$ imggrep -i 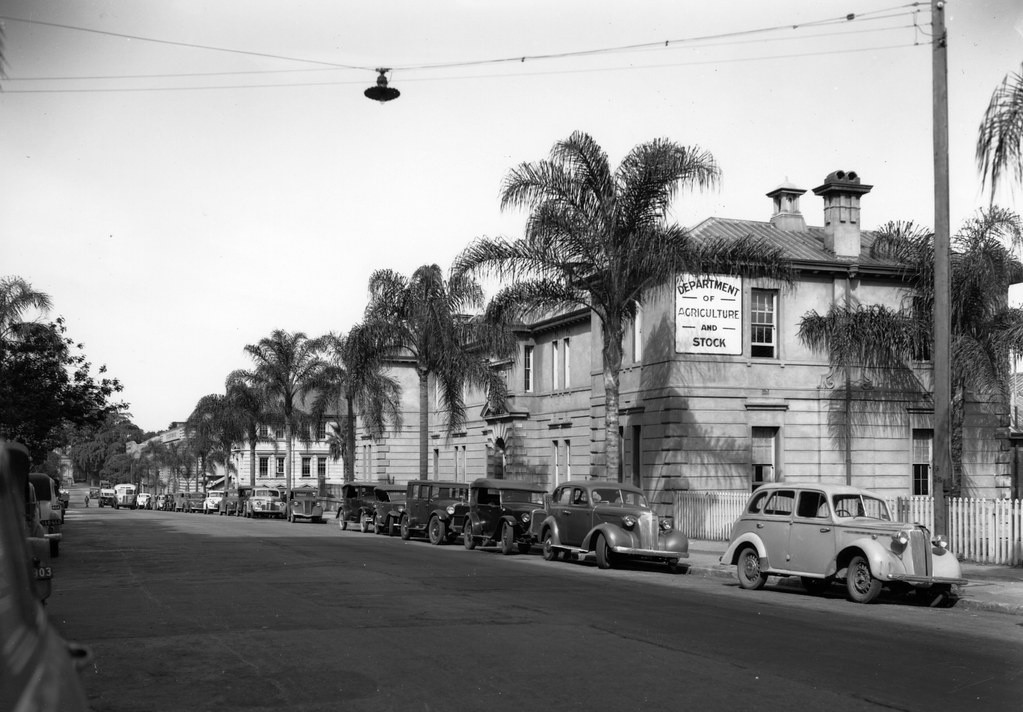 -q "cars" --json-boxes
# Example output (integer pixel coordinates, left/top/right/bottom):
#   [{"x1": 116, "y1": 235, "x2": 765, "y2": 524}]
[
  {"x1": 372, "y1": 486, "x2": 409, "y2": 535},
  {"x1": 527, "y1": 480, "x2": 690, "y2": 572},
  {"x1": 399, "y1": 480, "x2": 470, "y2": 545},
  {"x1": 288, "y1": 483, "x2": 323, "y2": 522},
  {"x1": 81, "y1": 478, "x2": 262, "y2": 517},
  {"x1": 719, "y1": 482, "x2": 967, "y2": 608},
  {"x1": 0, "y1": 438, "x2": 97, "y2": 712},
  {"x1": 452, "y1": 478, "x2": 548, "y2": 558},
  {"x1": 247, "y1": 488, "x2": 286, "y2": 519},
  {"x1": 335, "y1": 481, "x2": 385, "y2": 533}
]
